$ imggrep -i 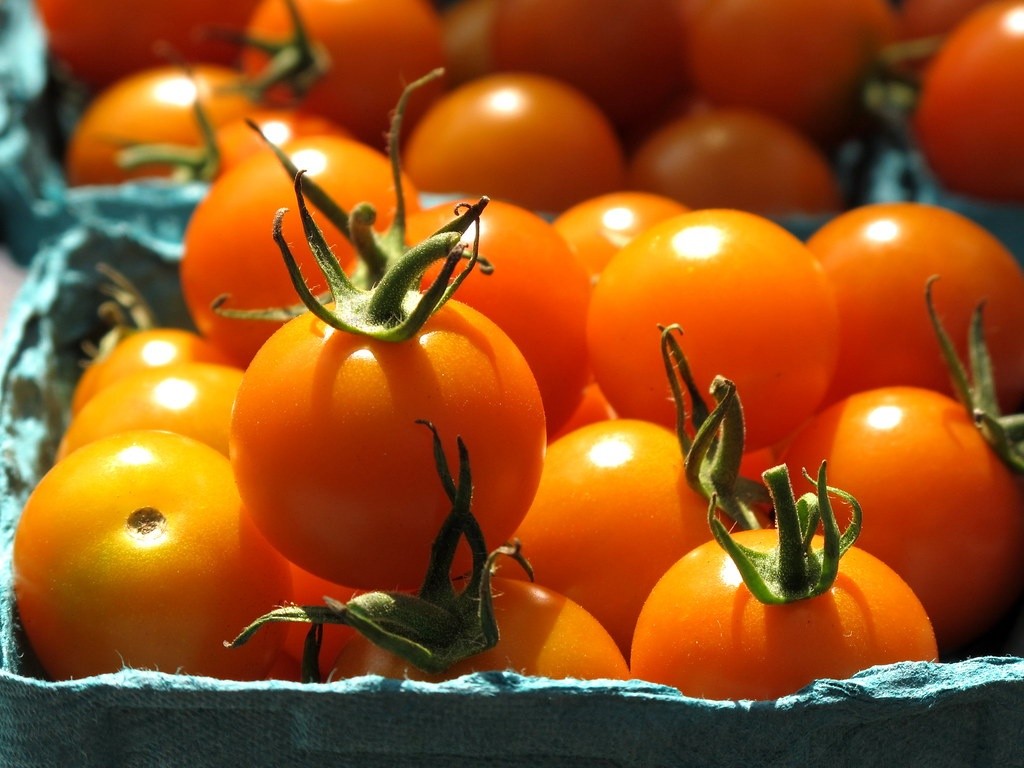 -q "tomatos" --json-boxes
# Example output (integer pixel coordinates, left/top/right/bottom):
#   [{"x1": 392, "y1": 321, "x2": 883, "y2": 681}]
[{"x1": 15, "y1": 0, "x2": 1024, "y2": 701}]
